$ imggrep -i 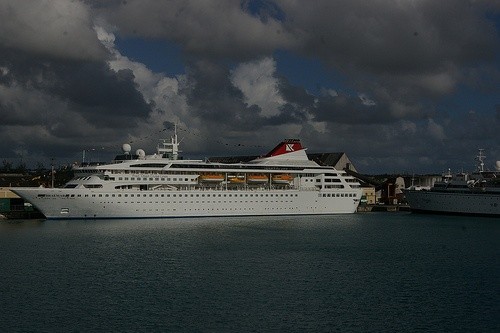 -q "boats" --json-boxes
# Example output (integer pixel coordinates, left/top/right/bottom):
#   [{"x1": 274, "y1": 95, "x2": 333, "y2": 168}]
[{"x1": 0, "y1": 122, "x2": 499, "y2": 219}]
